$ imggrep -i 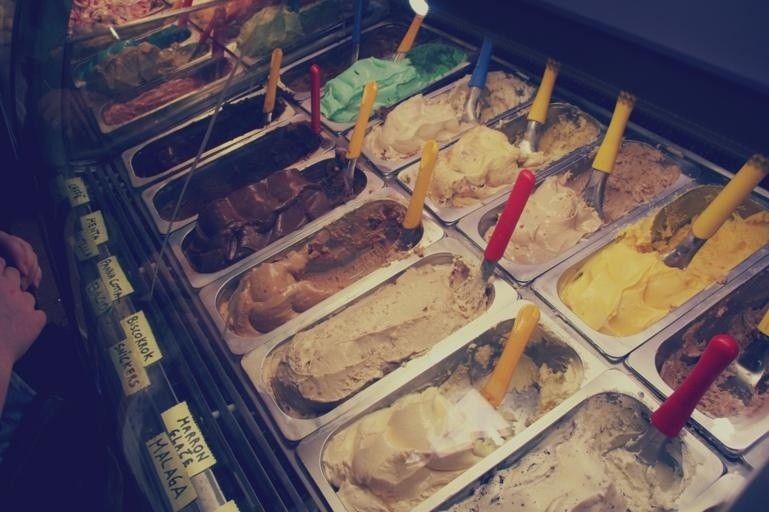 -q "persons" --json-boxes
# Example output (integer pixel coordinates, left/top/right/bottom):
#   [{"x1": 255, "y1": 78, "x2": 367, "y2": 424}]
[{"x1": 0, "y1": 230, "x2": 49, "y2": 421}]
